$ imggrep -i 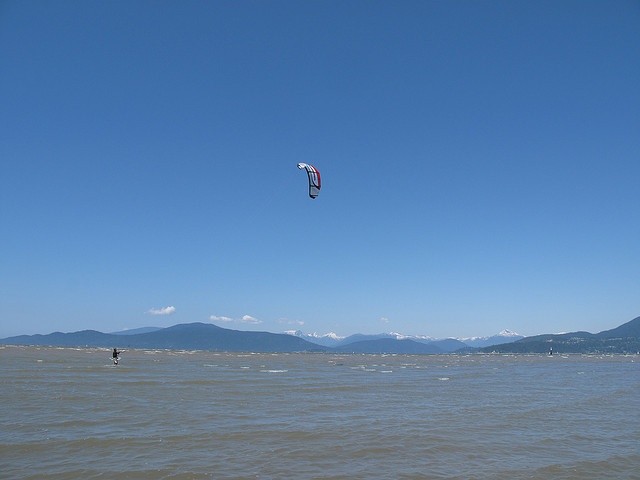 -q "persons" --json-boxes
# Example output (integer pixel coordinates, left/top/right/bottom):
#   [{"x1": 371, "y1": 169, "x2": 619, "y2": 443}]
[{"x1": 111, "y1": 348, "x2": 120, "y2": 366}]
